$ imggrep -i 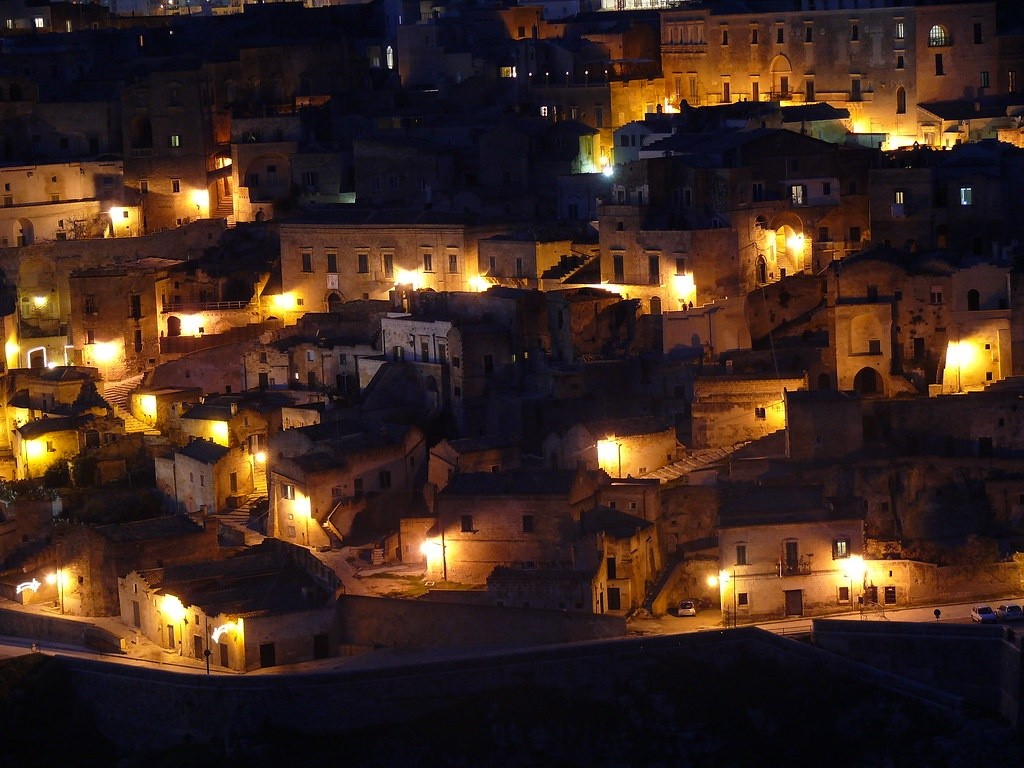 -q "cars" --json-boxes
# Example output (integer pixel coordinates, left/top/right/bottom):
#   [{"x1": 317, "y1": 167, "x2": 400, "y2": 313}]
[
  {"x1": 971, "y1": 605, "x2": 998, "y2": 624},
  {"x1": 996, "y1": 603, "x2": 1024, "y2": 622},
  {"x1": 676, "y1": 599, "x2": 697, "y2": 617}
]
